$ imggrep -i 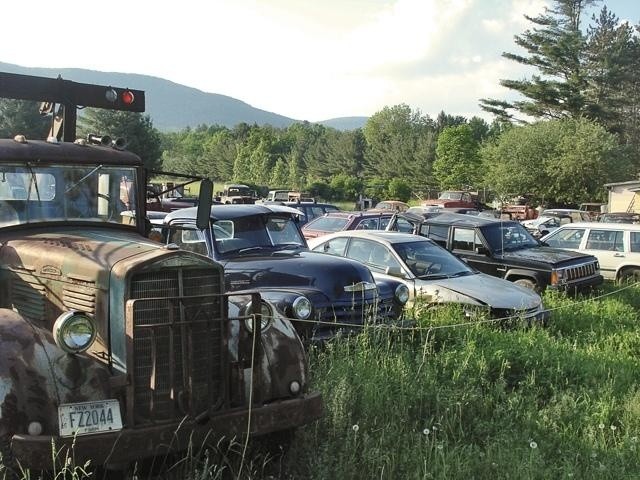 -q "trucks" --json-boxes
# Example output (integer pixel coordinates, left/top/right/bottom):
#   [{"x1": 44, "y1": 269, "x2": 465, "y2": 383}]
[{"x1": 0, "y1": 72, "x2": 324, "y2": 474}]
